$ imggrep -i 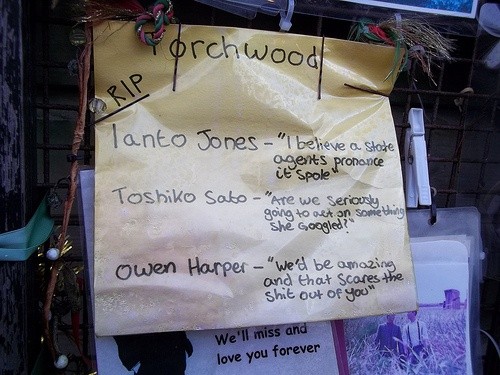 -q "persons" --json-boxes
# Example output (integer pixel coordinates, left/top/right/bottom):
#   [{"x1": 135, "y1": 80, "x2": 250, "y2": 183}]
[
  {"x1": 374, "y1": 313, "x2": 405, "y2": 363},
  {"x1": 402, "y1": 309, "x2": 432, "y2": 364}
]
